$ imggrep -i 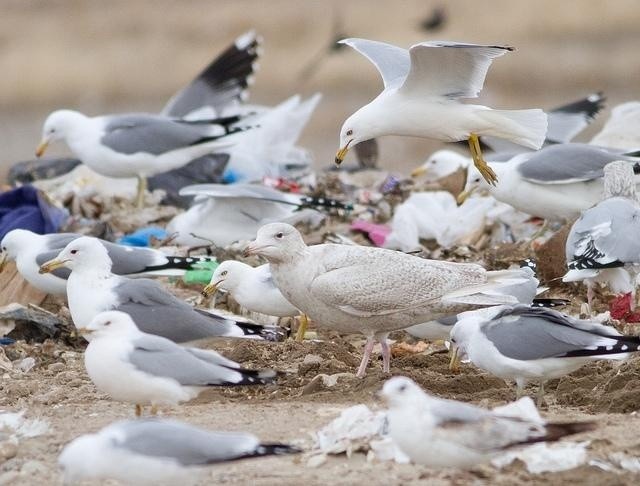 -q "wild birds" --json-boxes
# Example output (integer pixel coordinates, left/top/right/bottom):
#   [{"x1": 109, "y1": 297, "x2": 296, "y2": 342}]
[
  {"x1": 376, "y1": 373, "x2": 599, "y2": 485},
  {"x1": 55, "y1": 413, "x2": 306, "y2": 486},
  {"x1": 1, "y1": 28, "x2": 640, "y2": 418}
]
